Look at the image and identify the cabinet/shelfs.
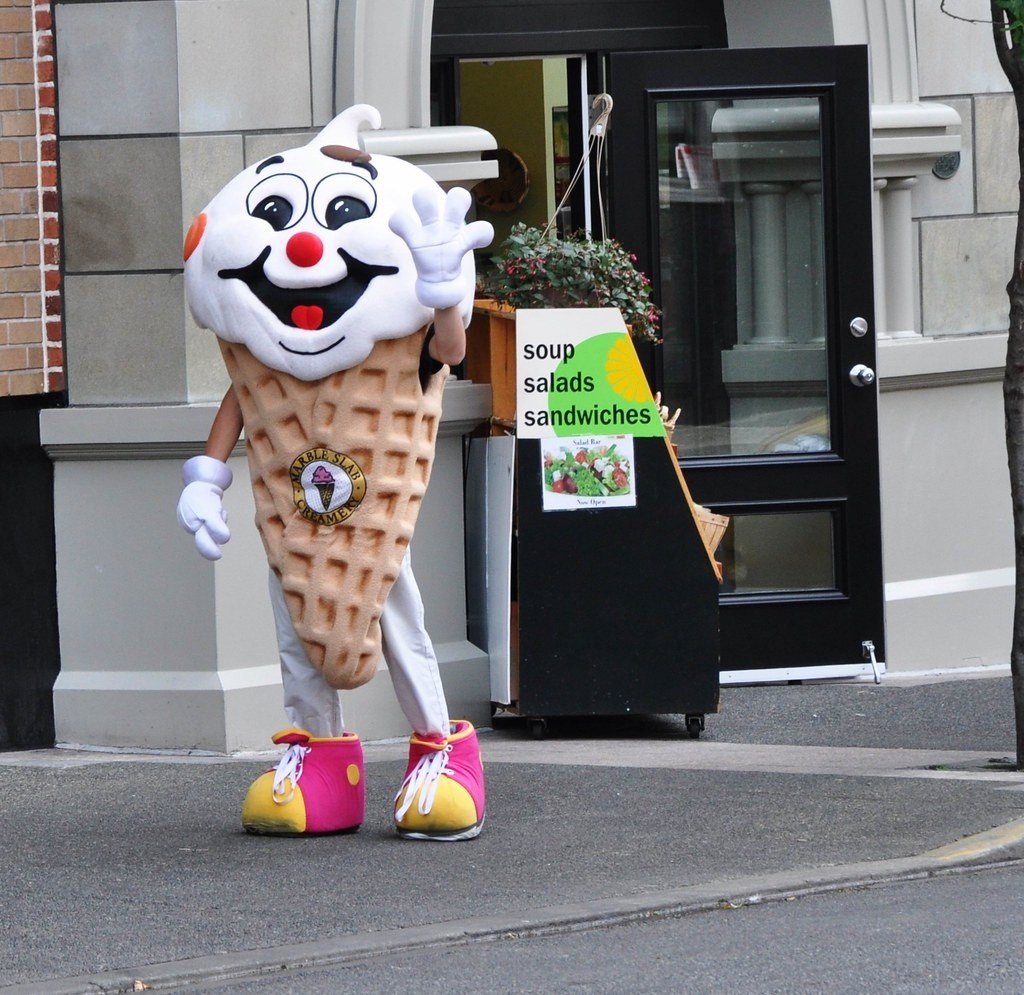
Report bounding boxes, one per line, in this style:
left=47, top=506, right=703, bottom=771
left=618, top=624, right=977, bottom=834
left=470, top=297, right=731, bottom=742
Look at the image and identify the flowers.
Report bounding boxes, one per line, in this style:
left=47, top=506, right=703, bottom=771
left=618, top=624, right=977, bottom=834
left=488, top=223, right=663, bottom=344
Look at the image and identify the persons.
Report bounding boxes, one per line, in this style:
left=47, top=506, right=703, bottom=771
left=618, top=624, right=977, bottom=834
left=174, top=100, right=497, bottom=845
